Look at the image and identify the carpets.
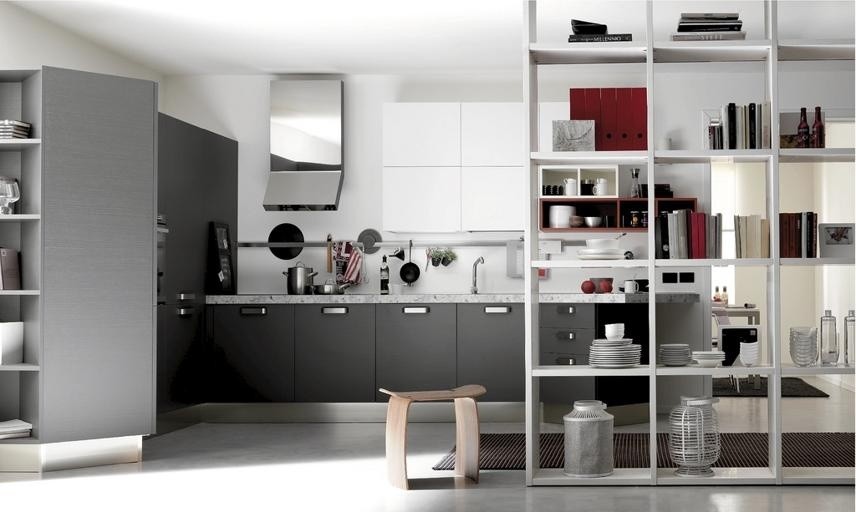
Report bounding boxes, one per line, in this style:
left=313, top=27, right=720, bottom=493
left=713, top=377, right=830, bottom=398
left=431, top=433, right=854, bottom=471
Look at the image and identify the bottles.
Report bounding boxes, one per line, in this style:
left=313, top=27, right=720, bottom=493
left=630, top=211, right=639, bottom=226
left=844, top=310, right=855, bottom=367
left=798, top=107, right=809, bottom=149
left=722, top=287, right=728, bottom=307
left=812, top=107, right=825, bottom=149
left=629, top=168, right=641, bottom=199
left=661, top=211, right=669, bottom=225
left=820, top=310, right=838, bottom=367
left=714, top=286, right=722, bottom=302
left=641, top=211, right=648, bottom=228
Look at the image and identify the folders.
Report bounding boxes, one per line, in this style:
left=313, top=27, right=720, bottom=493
left=632, top=88, right=647, bottom=150
left=616, top=88, right=632, bottom=150
left=570, top=89, right=585, bottom=120
left=585, top=89, right=601, bottom=150
left=601, top=88, right=616, bottom=150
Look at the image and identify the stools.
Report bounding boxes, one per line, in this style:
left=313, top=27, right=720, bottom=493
left=378, top=384, right=487, bottom=490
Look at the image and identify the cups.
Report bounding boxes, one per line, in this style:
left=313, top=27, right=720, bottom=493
left=565, top=179, right=577, bottom=196
left=593, top=184, right=609, bottom=196
left=624, top=281, right=639, bottom=294
left=596, top=178, right=607, bottom=185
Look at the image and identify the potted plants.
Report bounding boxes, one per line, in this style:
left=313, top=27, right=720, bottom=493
left=441, top=249, right=457, bottom=267
left=431, top=250, right=445, bottom=266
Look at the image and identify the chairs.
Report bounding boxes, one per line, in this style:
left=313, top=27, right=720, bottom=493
left=711, top=307, right=741, bottom=393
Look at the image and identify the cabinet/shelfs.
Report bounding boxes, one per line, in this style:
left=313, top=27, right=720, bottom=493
left=538, top=303, right=595, bottom=403
left=539, top=198, right=697, bottom=232
left=525, top=1, right=855, bottom=486
left=375, top=304, right=523, bottom=403
left=539, top=165, right=618, bottom=198
left=213, top=304, right=375, bottom=403
left=0, top=66, right=156, bottom=473
left=156, top=111, right=237, bottom=412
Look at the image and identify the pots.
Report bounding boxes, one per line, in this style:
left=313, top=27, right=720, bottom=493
left=311, top=282, right=350, bottom=296
left=282, top=261, right=317, bottom=294
left=400, top=241, right=421, bottom=285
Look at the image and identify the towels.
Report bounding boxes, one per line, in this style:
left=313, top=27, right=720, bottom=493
left=333, top=241, right=364, bottom=286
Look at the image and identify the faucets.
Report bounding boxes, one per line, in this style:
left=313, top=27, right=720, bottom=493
left=471, top=256, right=485, bottom=294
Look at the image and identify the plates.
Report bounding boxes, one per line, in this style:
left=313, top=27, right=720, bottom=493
left=579, top=254, right=625, bottom=260
left=692, top=351, right=726, bottom=367
left=588, top=339, right=641, bottom=368
left=659, top=343, right=691, bottom=366
left=577, top=248, right=625, bottom=255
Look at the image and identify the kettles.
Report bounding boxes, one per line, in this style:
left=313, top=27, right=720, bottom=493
left=1, top=176, right=22, bottom=215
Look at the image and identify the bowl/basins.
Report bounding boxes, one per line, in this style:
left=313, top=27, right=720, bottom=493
left=584, top=216, right=603, bottom=227
left=586, top=239, right=621, bottom=248
left=569, top=216, right=583, bottom=226
left=603, top=324, right=624, bottom=339
left=790, top=326, right=818, bottom=367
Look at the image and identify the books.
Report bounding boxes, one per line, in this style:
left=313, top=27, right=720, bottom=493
left=672, top=12, right=746, bottom=41
left=0, top=119, right=31, bottom=139
left=778, top=212, right=818, bottom=259
left=654, top=208, right=771, bottom=260
left=707, top=102, right=771, bottom=150
left=0, top=419, right=32, bottom=440
left=569, top=34, right=633, bottom=42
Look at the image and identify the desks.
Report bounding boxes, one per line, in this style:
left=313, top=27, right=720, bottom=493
left=714, top=308, right=760, bottom=325
